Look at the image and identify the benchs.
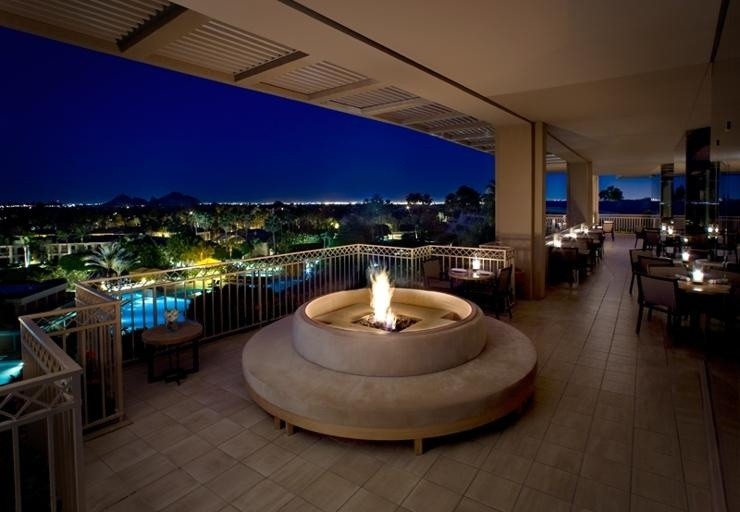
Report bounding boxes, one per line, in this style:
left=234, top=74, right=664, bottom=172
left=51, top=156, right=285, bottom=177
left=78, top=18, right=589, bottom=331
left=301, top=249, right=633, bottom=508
left=242, top=311, right=538, bottom=456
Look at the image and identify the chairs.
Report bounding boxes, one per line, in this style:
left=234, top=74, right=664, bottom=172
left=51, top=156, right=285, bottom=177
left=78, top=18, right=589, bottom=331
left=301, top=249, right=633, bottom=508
left=546, top=220, right=739, bottom=347
left=420, top=255, right=514, bottom=320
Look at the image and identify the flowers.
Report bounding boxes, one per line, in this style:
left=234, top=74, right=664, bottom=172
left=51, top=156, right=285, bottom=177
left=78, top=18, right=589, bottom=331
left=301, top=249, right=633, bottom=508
left=164, top=310, right=179, bottom=322
